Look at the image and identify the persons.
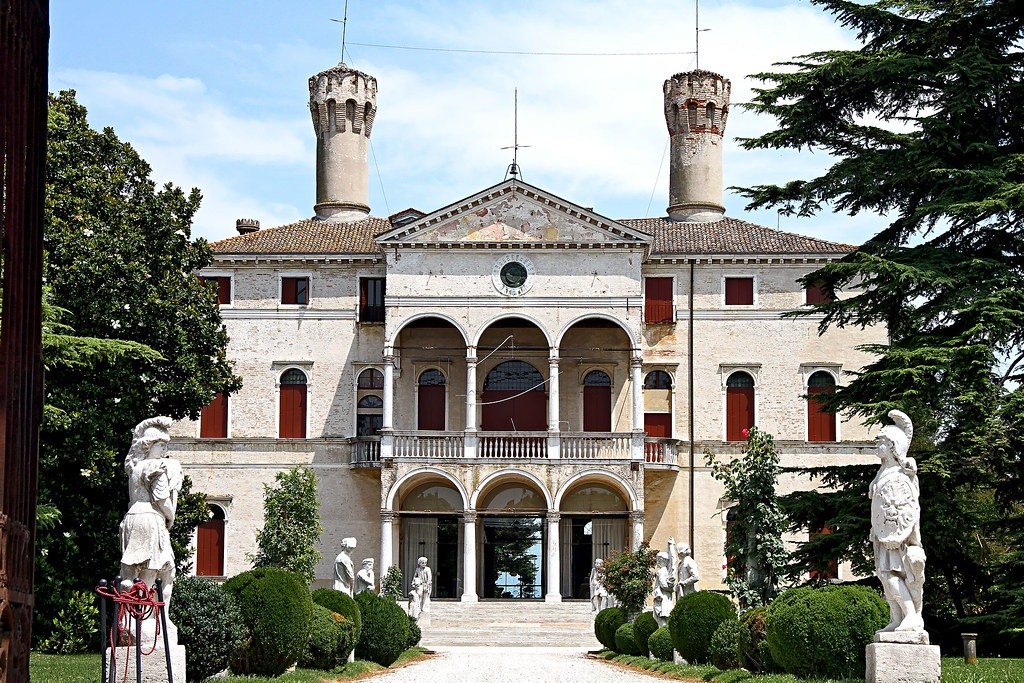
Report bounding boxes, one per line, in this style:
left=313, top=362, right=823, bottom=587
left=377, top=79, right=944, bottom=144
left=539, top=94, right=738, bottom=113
left=589, top=559, right=607, bottom=614
left=119, top=417, right=184, bottom=630
left=869, top=426, right=922, bottom=630
left=332, top=538, right=357, bottom=662
left=412, top=557, right=432, bottom=611
left=356, top=558, right=376, bottom=594
left=676, top=543, right=699, bottom=600
left=653, top=551, right=672, bottom=627
